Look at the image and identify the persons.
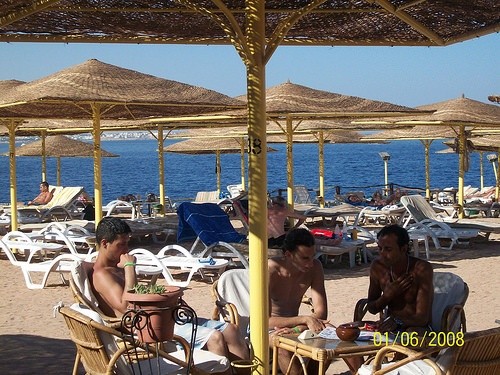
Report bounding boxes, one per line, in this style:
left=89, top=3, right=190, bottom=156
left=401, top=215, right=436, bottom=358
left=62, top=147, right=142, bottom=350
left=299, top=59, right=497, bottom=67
left=268, top=207, right=306, bottom=248
left=367, top=223, right=433, bottom=335
left=24, top=182, right=53, bottom=205
left=88, top=216, right=251, bottom=375
left=345, top=194, right=389, bottom=211
left=267, top=228, right=330, bottom=375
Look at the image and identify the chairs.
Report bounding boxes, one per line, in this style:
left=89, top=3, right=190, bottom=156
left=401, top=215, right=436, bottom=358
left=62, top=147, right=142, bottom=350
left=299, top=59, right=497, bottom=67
left=211, top=269, right=313, bottom=375
left=354, top=271, right=469, bottom=337
left=0, top=183, right=500, bottom=290
left=357, top=325, right=500, bottom=375
left=52, top=260, right=232, bottom=375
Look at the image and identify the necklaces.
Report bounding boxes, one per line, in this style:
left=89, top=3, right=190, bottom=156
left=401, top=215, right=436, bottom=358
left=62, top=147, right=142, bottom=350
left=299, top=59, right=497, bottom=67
left=390, top=253, right=409, bottom=281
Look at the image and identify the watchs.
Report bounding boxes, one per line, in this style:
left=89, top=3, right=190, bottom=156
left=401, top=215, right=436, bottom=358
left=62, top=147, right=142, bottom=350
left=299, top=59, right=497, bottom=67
left=394, top=316, right=405, bottom=331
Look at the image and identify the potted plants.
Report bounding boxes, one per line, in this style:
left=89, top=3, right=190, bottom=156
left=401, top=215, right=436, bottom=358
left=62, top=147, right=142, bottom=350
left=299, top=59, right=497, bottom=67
left=124, top=283, right=184, bottom=342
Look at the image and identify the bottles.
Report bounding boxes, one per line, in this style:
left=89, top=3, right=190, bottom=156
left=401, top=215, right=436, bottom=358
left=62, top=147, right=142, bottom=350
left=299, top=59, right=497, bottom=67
left=335, top=224, right=340, bottom=240
left=221, top=192, right=224, bottom=199
left=336, top=324, right=360, bottom=342
left=356, top=248, right=362, bottom=265
left=343, top=222, right=347, bottom=240
left=352, top=227, right=357, bottom=240
left=375, top=217, right=381, bottom=224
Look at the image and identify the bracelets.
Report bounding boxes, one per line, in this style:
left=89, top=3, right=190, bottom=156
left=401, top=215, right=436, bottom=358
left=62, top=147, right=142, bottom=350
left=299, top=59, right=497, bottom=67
left=294, top=326, right=300, bottom=333
left=123, top=261, right=136, bottom=266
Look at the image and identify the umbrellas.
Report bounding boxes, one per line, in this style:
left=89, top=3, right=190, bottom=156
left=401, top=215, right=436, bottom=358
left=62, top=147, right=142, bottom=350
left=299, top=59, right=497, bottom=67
left=0, top=0, right=500, bottom=375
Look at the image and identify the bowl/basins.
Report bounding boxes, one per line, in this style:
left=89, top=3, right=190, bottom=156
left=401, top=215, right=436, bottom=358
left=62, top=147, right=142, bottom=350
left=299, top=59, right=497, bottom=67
left=380, top=221, right=385, bottom=224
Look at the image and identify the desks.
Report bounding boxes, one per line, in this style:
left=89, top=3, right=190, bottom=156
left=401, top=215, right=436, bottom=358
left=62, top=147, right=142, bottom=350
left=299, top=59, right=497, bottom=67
left=273, top=330, right=430, bottom=375
left=131, top=200, right=160, bottom=220
